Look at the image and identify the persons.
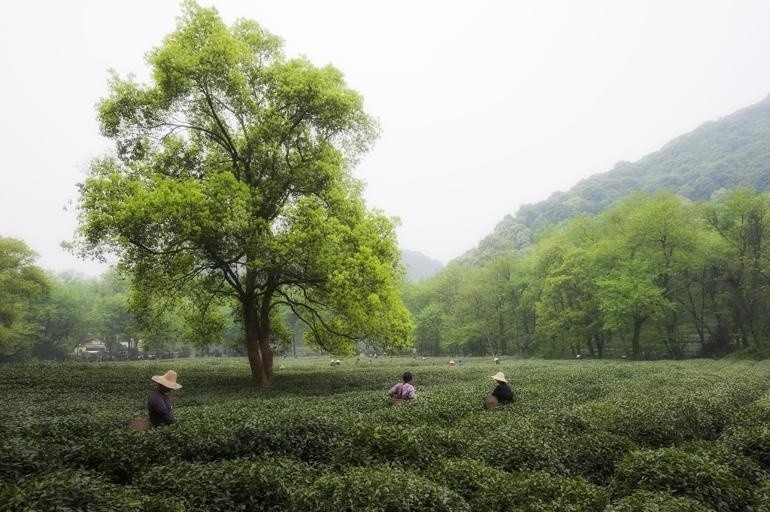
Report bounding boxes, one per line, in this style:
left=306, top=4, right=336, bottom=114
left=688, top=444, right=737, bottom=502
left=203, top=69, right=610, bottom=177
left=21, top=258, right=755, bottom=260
left=388, top=371, right=418, bottom=400
left=323, top=348, right=585, bottom=366
left=490, top=372, right=514, bottom=404
left=147, top=370, right=181, bottom=428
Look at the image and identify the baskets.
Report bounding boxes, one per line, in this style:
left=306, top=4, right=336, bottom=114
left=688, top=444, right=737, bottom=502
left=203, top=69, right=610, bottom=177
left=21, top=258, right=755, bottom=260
left=485, top=397, right=499, bottom=409
left=387, top=398, right=406, bottom=407
left=129, top=419, right=152, bottom=432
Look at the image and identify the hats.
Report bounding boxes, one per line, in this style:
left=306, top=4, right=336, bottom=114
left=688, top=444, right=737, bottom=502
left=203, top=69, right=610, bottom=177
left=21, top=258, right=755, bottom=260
left=491, top=372, right=507, bottom=382
left=151, top=370, right=182, bottom=390
